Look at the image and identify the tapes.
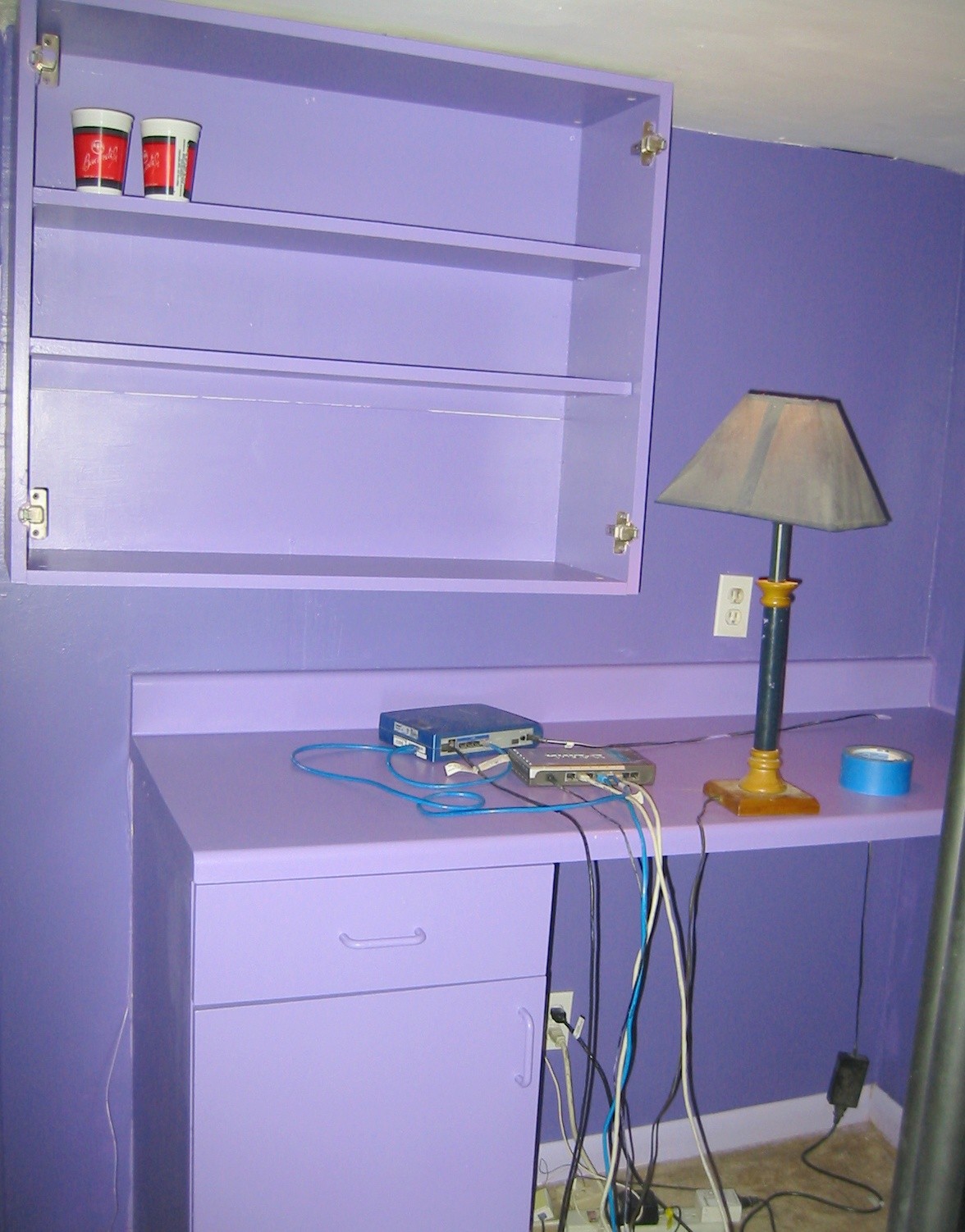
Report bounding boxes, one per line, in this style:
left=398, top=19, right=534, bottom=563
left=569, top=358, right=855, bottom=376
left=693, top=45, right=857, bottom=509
left=838, top=743, right=915, bottom=798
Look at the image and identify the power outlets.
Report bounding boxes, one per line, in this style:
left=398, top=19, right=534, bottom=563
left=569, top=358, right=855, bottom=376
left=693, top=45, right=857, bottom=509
left=713, top=573, right=753, bottom=638
left=546, top=991, right=573, bottom=1050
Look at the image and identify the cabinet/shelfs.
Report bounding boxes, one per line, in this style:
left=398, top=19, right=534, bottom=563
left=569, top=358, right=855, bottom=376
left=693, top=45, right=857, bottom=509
left=0, top=0, right=674, bottom=594
left=193, top=864, right=555, bottom=1231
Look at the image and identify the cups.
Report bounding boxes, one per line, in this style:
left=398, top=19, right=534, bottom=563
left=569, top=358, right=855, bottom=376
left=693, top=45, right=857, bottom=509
left=141, top=117, right=203, bottom=201
left=71, top=107, right=134, bottom=196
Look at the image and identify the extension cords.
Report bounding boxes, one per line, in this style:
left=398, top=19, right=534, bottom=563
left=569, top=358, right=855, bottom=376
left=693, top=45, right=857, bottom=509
left=563, top=1207, right=727, bottom=1232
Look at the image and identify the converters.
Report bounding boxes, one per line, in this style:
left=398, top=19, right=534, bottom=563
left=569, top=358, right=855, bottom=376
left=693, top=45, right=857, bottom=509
left=827, top=1052, right=869, bottom=1106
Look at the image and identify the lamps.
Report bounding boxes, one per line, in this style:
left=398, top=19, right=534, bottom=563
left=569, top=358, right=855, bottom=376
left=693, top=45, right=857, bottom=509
left=654, top=392, right=891, bottom=819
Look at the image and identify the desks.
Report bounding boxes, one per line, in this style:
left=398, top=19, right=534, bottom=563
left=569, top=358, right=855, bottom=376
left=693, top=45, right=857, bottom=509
left=132, top=708, right=965, bottom=1232
